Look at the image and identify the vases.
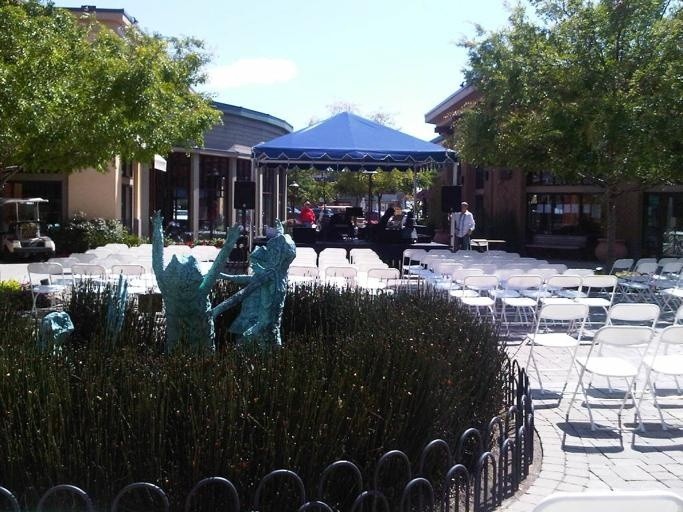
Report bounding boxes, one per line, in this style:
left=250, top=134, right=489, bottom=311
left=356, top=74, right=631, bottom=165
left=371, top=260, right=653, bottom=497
left=592, top=236, right=628, bottom=263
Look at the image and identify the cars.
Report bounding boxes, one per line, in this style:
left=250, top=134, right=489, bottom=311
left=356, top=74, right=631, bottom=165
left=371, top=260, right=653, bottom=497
left=545, top=223, right=607, bottom=238
left=172, top=210, right=188, bottom=221
left=235, top=205, right=422, bottom=227
left=661, top=230, right=682, bottom=257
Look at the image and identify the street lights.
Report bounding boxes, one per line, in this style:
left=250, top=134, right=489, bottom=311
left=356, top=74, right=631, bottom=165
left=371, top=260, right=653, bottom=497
left=287, top=180, right=300, bottom=218
left=361, top=170, right=378, bottom=213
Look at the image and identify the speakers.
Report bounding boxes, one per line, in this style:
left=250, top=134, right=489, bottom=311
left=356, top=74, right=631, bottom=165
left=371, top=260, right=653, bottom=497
left=441, top=185, right=462, bottom=212
left=234, top=182, right=255, bottom=209
left=292, top=228, right=316, bottom=244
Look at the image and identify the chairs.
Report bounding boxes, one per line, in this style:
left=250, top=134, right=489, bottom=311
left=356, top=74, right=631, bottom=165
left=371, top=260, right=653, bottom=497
left=287, top=247, right=399, bottom=298
left=400, top=247, right=546, bottom=336
left=24, top=241, right=221, bottom=300
left=525, top=258, right=683, bottom=432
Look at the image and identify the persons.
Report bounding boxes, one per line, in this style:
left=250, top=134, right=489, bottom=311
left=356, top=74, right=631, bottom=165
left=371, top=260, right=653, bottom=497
left=448, top=201, right=475, bottom=251
left=299, top=200, right=315, bottom=228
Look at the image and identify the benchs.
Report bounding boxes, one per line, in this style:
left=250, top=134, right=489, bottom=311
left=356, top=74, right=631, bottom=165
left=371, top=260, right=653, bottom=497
left=524, top=233, right=587, bottom=259
